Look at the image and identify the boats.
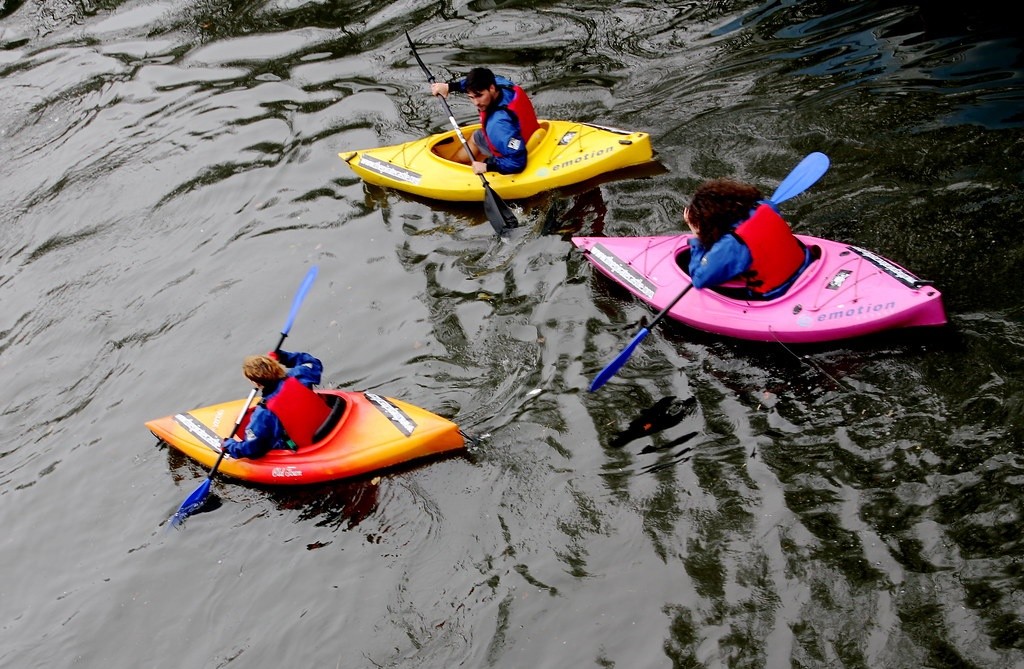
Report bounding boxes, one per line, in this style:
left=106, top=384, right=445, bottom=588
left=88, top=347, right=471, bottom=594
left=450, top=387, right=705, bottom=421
left=144, top=390, right=463, bottom=485
left=574, top=236, right=947, bottom=342
left=337, top=119, right=659, bottom=201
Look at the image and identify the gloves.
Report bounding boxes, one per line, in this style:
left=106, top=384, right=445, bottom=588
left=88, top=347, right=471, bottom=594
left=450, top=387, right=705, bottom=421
left=222, top=438, right=228, bottom=451
left=269, top=352, right=279, bottom=361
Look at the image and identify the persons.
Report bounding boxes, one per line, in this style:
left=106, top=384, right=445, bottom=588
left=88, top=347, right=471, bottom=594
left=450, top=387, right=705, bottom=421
left=219, top=350, right=332, bottom=460
left=432, top=68, right=540, bottom=176
left=683, top=180, right=810, bottom=301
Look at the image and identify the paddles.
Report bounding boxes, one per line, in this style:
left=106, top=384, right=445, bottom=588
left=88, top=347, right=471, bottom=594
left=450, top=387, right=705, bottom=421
left=165, top=265, right=318, bottom=534
left=587, top=150, right=831, bottom=392
left=403, top=27, right=520, bottom=234
